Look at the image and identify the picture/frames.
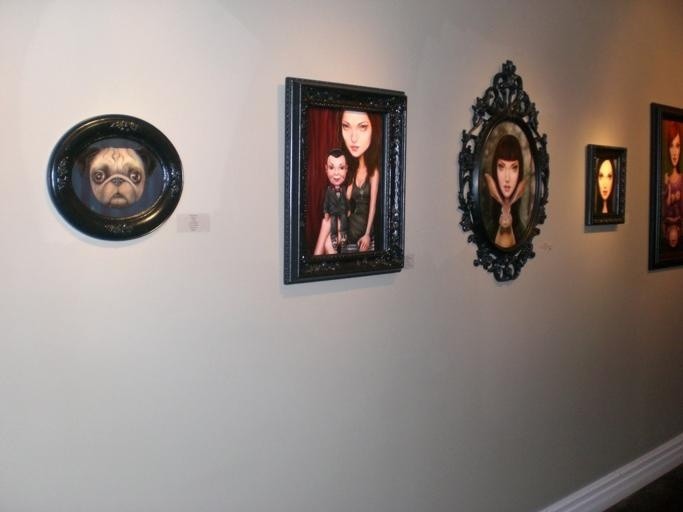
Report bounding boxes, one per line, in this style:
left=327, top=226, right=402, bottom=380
left=648, top=102, right=682, bottom=272
left=283, top=76, right=404, bottom=286
left=47, top=113, right=183, bottom=242
left=457, top=60, right=550, bottom=282
left=584, top=144, right=627, bottom=225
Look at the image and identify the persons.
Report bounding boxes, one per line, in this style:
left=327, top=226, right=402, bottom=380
left=663, top=123, right=683, bottom=248
left=322, top=147, right=352, bottom=248
left=596, top=159, right=616, bottom=213
left=314, top=110, right=379, bottom=255
left=485, top=134, right=530, bottom=249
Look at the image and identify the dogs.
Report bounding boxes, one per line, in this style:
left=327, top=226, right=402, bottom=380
left=75, top=143, right=158, bottom=209
